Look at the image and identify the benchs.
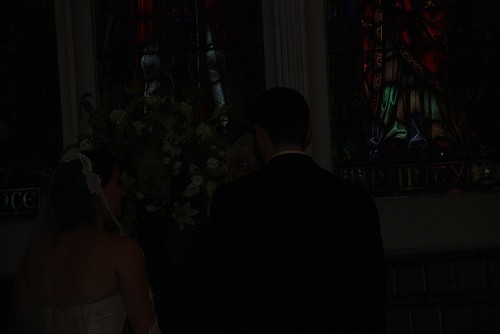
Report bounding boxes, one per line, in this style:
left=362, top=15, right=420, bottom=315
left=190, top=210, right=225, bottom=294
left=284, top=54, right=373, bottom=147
left=382, top=241, right=499, bottom=334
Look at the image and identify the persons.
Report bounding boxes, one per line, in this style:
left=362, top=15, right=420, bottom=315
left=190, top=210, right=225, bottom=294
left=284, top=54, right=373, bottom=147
left=199, top=86, right=387, bottom=334
left=13, top=149, right=161, bottom=334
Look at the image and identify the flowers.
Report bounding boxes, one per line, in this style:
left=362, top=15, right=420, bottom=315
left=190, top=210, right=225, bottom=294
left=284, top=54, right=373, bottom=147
left=66, top=89, right=241, bottom=236
